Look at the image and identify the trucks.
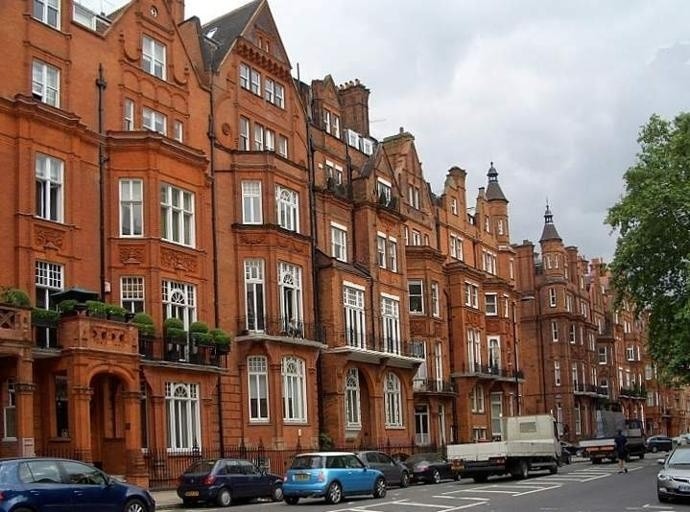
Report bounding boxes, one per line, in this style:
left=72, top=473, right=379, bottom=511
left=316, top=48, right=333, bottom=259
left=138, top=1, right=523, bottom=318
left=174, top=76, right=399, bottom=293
left=579, top=419, right=646, bottom=463
left=445, top=413, right=569, bottom=481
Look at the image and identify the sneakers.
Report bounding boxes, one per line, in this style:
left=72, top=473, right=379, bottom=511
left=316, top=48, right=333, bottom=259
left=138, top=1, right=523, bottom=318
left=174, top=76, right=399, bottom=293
left=618, top=467, right=627, bottom=473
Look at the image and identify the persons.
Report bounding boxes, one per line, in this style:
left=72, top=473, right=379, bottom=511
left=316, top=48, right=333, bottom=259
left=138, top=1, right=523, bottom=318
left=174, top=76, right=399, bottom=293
left=614, top=428, right=630, bottom=474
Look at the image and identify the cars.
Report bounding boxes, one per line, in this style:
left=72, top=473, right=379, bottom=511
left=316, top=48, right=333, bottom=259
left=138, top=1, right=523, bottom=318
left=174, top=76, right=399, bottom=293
left=656, top=441, right=690, bottom=504
left=1, top=456, right=158, bottom=512
left=280, top=451, right=461, bottom=503
left=644, top=433, right=690, bottom=453
left=178, top=457, right=285, bottom=507
left=559, top=447, right=572, bottom=466
